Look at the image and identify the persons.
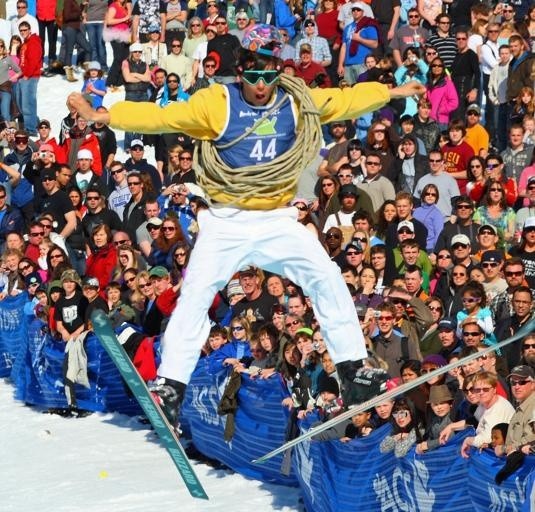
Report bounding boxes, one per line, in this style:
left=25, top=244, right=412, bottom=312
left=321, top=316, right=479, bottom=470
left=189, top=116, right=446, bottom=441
left=293, top=151, right=535, bottom=457
left=0, top=246, right=182, bottom=418
left=0, top=169, right=191, bottom=285
left=155, top=266, right=305, bottom=469
left=276, top=1, right=534, bottom=198
left=66, top=23, right=427, bottom=406
left=104, top=1, right=295, bottom=238
left=1, top=1, right=117, bottom=218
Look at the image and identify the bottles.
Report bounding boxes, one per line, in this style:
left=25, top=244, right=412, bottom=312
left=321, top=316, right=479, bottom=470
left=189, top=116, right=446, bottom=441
left=241, top=23, right=286, bottom=58
left=426, top=385, right=454, bottom=404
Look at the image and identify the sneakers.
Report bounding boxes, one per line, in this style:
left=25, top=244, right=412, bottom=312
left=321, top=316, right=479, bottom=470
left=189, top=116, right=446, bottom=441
left=146, top=375, right=187, bottom=438
left=336, top=359, right=390, bottom=407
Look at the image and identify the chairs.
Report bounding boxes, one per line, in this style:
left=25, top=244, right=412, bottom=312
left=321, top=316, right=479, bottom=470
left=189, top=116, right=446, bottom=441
left=146, top=375, right=187, bottom=438
left=336, top=359, right=390, bottom=407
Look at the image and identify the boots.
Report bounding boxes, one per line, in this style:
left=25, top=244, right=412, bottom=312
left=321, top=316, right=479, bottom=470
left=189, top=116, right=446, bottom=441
left=62, top=66, right=77, bottom=82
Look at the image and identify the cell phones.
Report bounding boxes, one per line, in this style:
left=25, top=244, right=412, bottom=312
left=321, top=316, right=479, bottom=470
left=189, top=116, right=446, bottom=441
left=373, top=310, right=381, bottom=317
left=312, top=342, right=320, bottom=351
left=173, top=184, right=185, bottom=194
left=38, top=152, right=47, bottom=159
left=409, top=56, right=418, bottom=62
left=502, top=3, right=509, bottom=9
left=7, top=129, right=15, bottom=133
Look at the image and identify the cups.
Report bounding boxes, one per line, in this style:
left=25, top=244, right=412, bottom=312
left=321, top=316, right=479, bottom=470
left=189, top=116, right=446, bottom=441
left=238, top=69, right=278, bottom=85
left=392, top=410, right=410, bottom=419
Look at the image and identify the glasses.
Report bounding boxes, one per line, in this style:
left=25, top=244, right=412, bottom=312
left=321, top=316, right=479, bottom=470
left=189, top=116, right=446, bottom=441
left=1, top=6, right=245, bottom=330
left=392, top=410, right=410, bottom=419
left=284, top=8, right=535, bottom=395
left=238, top=69, right=278, bottom=85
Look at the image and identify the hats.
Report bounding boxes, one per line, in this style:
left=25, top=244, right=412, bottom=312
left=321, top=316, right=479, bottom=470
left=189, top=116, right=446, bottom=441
left=241, top=23, right=286, bottom=58
left=331, top=121, right=345, bottom=126
left=345, top=242, right=362, bottom=250
left=146, top=217, right=163, bottom=227
left=129, top=139, right=144, bottom=147
left=506, top=365, right=533, bottom=381
left=82, top=276, right=99, bottom=287
left=481, top=250, right=501, bottom=262
left=300, top=43, right=311, bottom=52
left=426, top=385, right=454, bottom=404
left=148, top=266, right=167, bottom=278
left=86, top=61, right=101, bottom=70
left=523, top=217, right=533, bottom=228
left=397, top=221, right=414, bottom=232
left=379, top=109, right=393, bottom=122
left=450, top=234, right=470, bottom=248
left=339, top=184, right=359, bottom=198
left=466, top=104, right=480, bottom=114
left=478, top=224, right=497, bottom=236
left=77, top=149, right=93, bottom=160
left=37, top=119, right=49, bottom=128
left=436, top=317, right=456, bottom=329
left=39, top=144, right=53, bottom=152
